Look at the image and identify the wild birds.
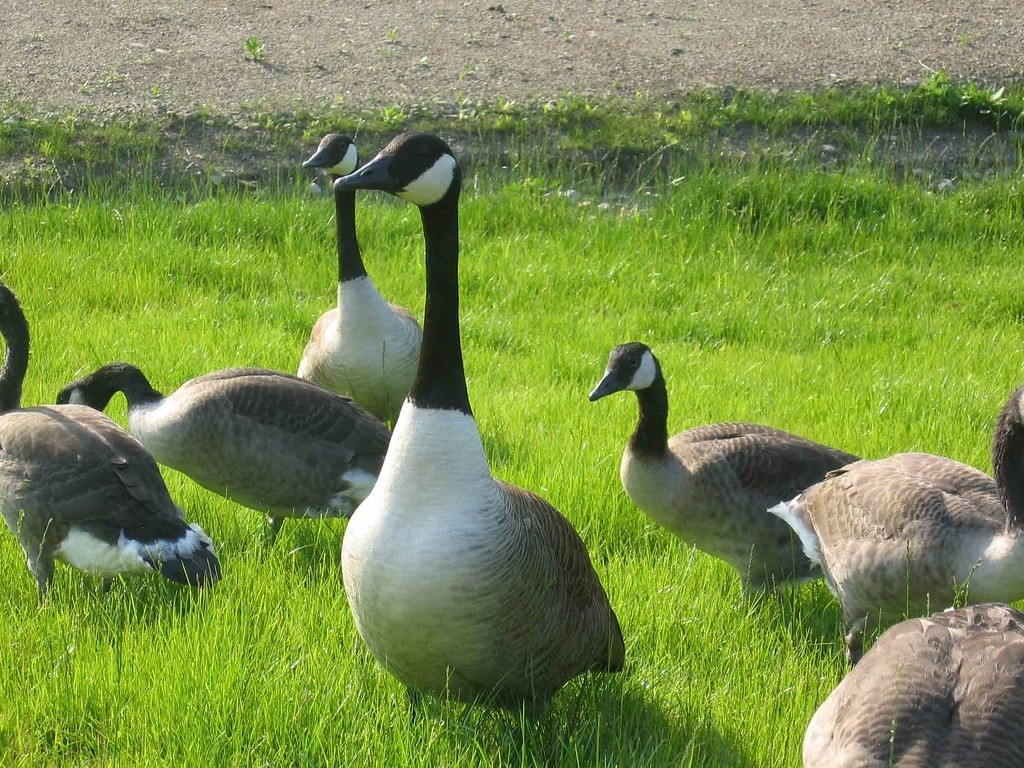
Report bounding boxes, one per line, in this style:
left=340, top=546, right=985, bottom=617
left=589, top=342, right=863, bottom=606
left=54, top=360, right=392, bottom=554
left=803, top=603, right=1024, bottom=768
left=331, top=131, right=626, bottom=708
left=763, top=385, right=1024, bottom=670
left=299, top=132, right=423, bottom=429
left=1, top=281, right=224, bottom=596
